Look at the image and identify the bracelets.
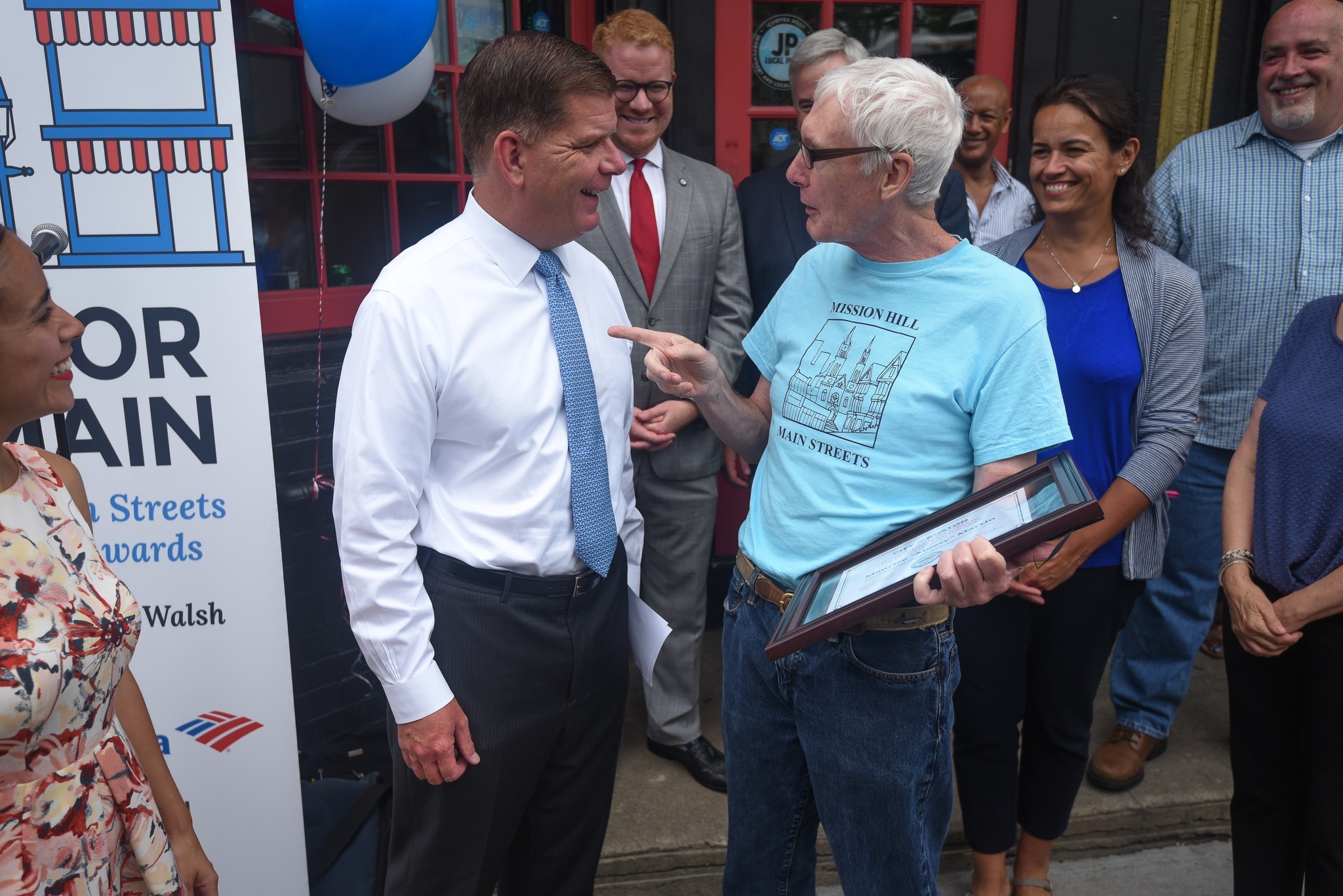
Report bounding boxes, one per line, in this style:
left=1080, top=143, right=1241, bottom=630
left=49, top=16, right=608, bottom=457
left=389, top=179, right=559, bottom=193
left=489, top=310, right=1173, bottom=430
left=1218, top=557, right=1255, bottom=580
left=1218, top=548, right=1255, bottom=569
left=1219, top=561, right=1254, bottom=587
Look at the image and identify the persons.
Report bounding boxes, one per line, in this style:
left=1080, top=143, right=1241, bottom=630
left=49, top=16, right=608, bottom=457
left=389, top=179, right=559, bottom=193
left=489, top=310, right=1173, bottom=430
left=952, top=74, right=1204, bottom=896
left=726, top=27, right=973, bottom=487
left=1086, top=0, right=1343, bottom=790
left=950, top=74, right=1037, bottom=247
left=1, top=225, right=218, bottom=895
left=329, top=33, right=645, bottom=896
left=611, top=57, right=1075, bottom=895
left=1218, top=293, right=1343, bottom=895
left=573, top=9, right=755, bottom=794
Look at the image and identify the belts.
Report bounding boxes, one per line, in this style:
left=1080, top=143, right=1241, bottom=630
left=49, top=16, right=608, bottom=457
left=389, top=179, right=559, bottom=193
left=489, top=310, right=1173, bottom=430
left=416, top=549, right=615, bottom=601
left=732, top=550, right=949, bottom=630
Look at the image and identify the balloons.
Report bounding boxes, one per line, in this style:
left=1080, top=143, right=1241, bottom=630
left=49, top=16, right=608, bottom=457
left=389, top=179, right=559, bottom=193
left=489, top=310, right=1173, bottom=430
left=293, top=0, right=439, bottom=87
left=302, top=37, right=436, bottom=124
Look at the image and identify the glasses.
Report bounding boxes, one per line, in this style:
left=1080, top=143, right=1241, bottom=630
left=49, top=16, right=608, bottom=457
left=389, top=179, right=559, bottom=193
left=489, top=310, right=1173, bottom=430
left=615, top=79, right=673, bottom=104
left=796, top=139, right=880, bottom=170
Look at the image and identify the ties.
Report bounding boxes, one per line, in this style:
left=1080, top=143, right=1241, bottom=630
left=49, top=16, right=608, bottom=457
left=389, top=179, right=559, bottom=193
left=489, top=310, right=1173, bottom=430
left=629, top=158, right=661, bottom=302
left=534, top=251, right=618, bottom=580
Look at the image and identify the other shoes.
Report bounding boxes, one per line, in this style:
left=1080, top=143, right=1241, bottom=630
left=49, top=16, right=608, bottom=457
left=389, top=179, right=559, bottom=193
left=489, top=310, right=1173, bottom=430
left=1201, top=634, right=1225, bottom=660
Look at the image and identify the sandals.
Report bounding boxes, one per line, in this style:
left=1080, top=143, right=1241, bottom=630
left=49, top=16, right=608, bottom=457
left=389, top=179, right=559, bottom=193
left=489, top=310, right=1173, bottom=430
left=1012, top=877, right=1053, bottom=896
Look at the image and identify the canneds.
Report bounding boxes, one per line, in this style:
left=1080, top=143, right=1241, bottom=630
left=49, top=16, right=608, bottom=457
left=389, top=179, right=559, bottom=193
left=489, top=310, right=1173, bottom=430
left=330, top=265, right=354, bottom=286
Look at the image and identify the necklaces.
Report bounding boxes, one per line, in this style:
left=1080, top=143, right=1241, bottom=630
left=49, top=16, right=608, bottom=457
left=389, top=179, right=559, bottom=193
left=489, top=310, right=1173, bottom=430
left=1041, top=233, right=1114, bottom=294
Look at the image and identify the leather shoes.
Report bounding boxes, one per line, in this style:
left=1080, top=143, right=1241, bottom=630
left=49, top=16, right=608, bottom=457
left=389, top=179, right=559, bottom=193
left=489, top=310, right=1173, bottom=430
left=648, top=736, right=727, bottom=793
left=1087, top=725, right=1167, bottom=791
